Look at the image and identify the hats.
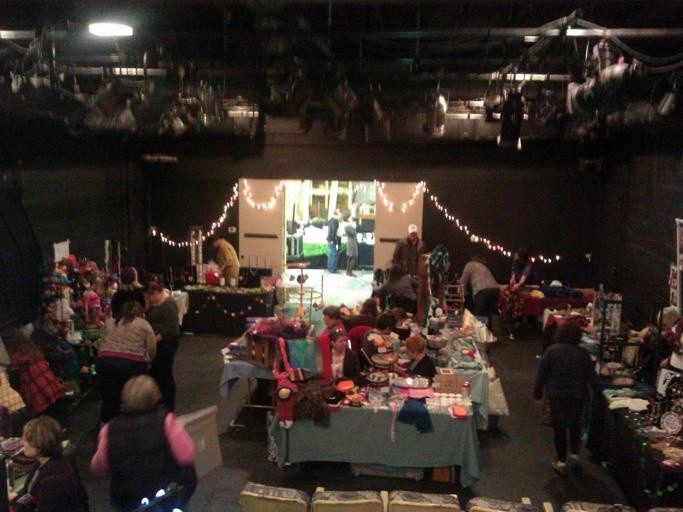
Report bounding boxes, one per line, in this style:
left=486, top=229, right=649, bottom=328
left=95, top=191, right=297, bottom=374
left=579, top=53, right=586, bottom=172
left=408, top=223, right=418, bottom=233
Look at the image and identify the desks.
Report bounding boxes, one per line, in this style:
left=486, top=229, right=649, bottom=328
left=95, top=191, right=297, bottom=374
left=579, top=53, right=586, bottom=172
left=1, top=236, right=683, bottom=511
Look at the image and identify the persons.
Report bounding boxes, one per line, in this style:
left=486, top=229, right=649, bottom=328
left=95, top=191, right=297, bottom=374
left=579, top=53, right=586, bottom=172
left=286, top=210, right=359, bottom=277
left=533, top=321, right=603, bottom=476
left=206, top=235, right=240, bottom=286
left=253, top=225, right=501, bottom=390
left=1, top=268, right=196, bottom=511
left=633, top=326, right=673, bottom=388
left=510, top=247, right=536, bottom=291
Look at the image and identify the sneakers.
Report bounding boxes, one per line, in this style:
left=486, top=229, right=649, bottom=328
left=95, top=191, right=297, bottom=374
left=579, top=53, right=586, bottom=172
left=552, top=460, right=567, bottom=476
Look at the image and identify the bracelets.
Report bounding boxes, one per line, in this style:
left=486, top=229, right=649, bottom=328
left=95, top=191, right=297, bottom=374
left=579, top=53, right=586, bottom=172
left=518, top=282, right=523, bottom=288
